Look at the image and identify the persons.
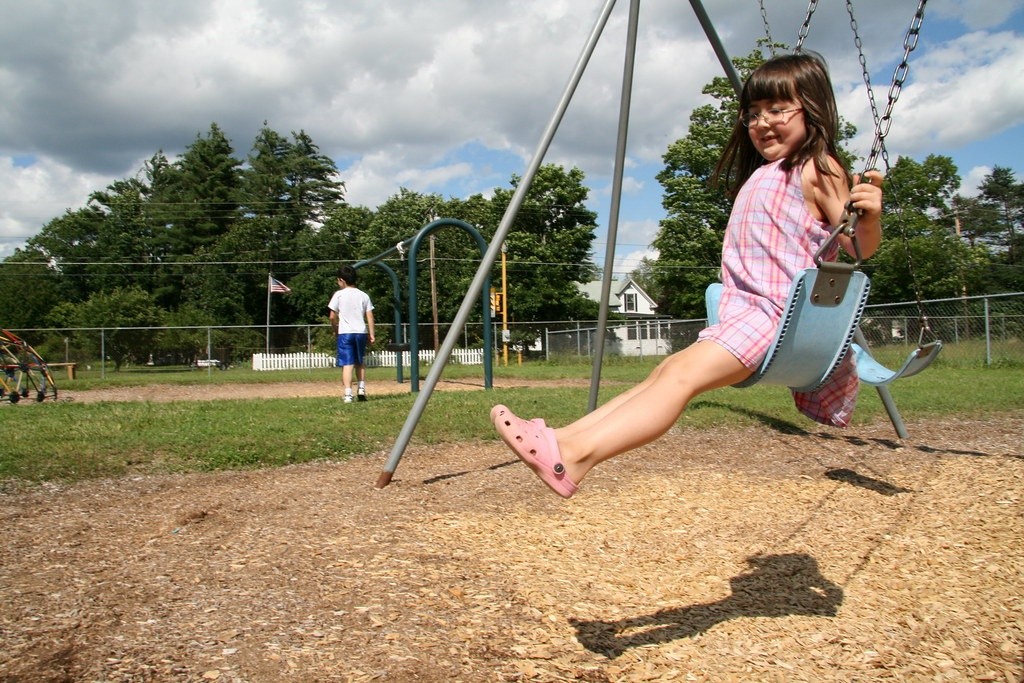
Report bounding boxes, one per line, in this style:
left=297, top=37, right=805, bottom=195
left=328, top=266, right=375, bottom=403
left=491, top=55, right=883, bottom=498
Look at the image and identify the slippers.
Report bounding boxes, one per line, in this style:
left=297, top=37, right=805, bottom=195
left=491, top=404, right=578, bottom=499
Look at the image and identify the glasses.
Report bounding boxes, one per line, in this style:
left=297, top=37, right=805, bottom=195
left=738, top=108, right=803, bottom=128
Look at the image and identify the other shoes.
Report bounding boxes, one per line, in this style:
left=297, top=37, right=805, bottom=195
left=357, top=387, right=369, bottom=400
left=344, top=395, right=355, bottom=404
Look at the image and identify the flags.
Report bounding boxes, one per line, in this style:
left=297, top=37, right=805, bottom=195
left=270, top=278, right=290, bottom=292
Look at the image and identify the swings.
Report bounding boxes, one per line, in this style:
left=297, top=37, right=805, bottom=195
left=702, top=0, right=932, bottom=395
left=384, top=241, right=423, bottom=352
left=756, top=0, right=947, bottom=388
